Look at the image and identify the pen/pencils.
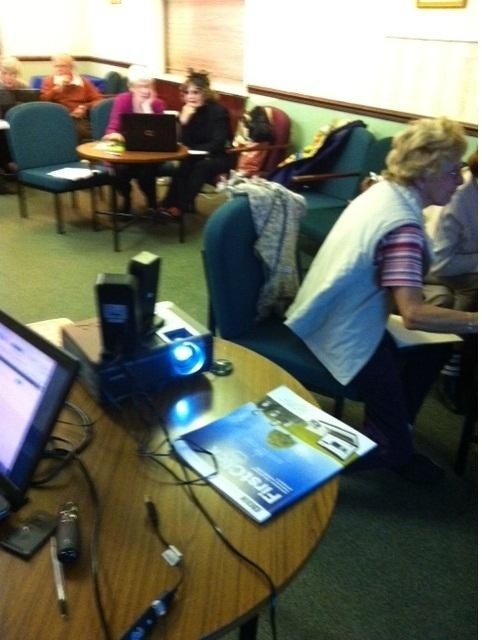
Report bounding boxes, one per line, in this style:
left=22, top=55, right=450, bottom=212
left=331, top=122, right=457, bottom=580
left=50, top=536, right=67, bottom=619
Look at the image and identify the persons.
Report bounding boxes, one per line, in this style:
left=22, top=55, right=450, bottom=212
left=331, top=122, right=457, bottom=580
left=422, top=150, right=478, bottom=417
left=38, top=52, right=104, bottom=139
left=253, top=109, right=477, bottom=514
left=155, top=72, right=236, bottom=225
left=0, top=55, right=30, bottom=89
left=103, top=64, right=164, bottom=213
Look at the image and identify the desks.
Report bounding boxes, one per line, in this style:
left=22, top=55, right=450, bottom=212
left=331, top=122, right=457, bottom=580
left=75, top=139, right=189, bottom=252
left=2, top=317, right=345, bottom=638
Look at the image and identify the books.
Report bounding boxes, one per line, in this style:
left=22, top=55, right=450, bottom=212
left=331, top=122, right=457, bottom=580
left=166, top=382, right=379, bottom=525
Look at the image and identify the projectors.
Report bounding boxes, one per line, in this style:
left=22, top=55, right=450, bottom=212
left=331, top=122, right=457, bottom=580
left=61, top=299, right=216, bottom=408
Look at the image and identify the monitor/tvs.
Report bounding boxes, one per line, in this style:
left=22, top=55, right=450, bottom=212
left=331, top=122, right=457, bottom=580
left=0, top=306, right=84, bottom=510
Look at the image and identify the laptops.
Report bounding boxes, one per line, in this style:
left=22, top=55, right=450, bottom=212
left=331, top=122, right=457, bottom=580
left=117, top=112, right=182, bottom=154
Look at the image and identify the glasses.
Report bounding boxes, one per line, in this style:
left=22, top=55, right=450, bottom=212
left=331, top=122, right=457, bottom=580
left=442, top=161, right=468, bottom=180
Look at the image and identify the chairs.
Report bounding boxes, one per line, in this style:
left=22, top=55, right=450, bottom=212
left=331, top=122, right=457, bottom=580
left=291, top=125, right=395, bottom=286
left=224, top=105, right=289, bottom=183
left=4, top=100, right=109, bottom=234
left=199, top=192, right=361, bottom=420
left=89, top=98, right=176, bottom=200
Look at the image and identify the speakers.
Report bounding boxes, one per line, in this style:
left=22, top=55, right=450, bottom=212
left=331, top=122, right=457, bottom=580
left=95, top=271, right=145, bottom=355
left=129, top=251, right=165, bottom=335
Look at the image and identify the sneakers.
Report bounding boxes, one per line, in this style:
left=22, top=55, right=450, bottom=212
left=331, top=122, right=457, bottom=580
left=147, top=206, right=181, bottom=219
